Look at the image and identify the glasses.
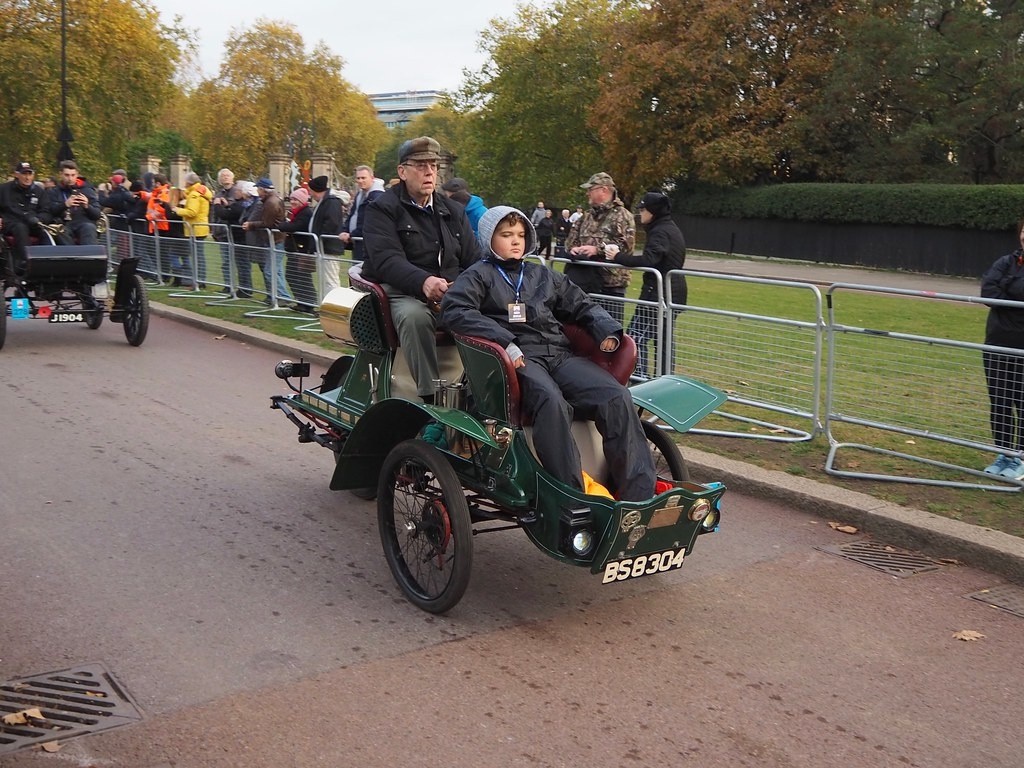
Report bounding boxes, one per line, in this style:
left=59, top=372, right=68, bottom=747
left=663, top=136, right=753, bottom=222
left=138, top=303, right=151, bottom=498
left=403, top=162, right=441, bottom=171
left=589, top=186, right=602, bottom=191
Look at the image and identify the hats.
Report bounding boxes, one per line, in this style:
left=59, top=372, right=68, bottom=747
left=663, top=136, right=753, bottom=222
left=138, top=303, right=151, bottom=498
left=252, top=179, right=274, bottom=189
left=14, top=163, right=33, bottom=173
left=398, top=136, right=442, bottom=164
left=579, top=173, right=615, bottom=189
left=112, top=169, right=127, bottom=176
left=290, top=188, right=310, bottom=205
left=184, top=173, right=201, bottom=184
left=635, top=194, right=671, bottom=217
left=441, top=178, right=468, bottom=193
left=309, top=176, right=328, bottom=191
left=130, top=180, right=145, bottom=192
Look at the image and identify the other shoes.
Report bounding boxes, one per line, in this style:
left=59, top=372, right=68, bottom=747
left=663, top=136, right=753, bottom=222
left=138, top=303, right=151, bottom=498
left=220, top=287, right=230, bottom=293
left=172, top=277, right=182, bottom=286
left=235, top=289, right=253, bottom=297
left=294, top=303, right=315, bottom=313
left=199, top=283, right=206, bottom=289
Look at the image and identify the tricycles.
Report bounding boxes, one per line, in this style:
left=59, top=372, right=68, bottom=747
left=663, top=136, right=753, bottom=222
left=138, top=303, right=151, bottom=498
left=270, top=259, right=729, bottom=616
left=0, top=213, right=151, bottom=352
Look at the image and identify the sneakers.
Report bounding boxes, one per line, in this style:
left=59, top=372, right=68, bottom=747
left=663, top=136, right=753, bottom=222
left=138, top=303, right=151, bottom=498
left=984, top=453, right=1013, bottom=475
left=1000, top=459, right=1024, bottom=480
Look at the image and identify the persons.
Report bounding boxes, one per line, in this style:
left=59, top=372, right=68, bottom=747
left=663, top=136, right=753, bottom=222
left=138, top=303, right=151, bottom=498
left=603, top=192, right=686, bottom=382
left=564, top=172, right=636, bottom=325
left=530, top=202, right=583, bottom=262
left=441, top=205, right=658, bottom=507
left=280, top=166, right=490, bottom=316
left=362, top=137, right=497, bottom=437
left=981, top=219, right=1024, bottom=481
left=98, top=168, right=291, bottom=305
left=0, top=159, right=101, bottom=273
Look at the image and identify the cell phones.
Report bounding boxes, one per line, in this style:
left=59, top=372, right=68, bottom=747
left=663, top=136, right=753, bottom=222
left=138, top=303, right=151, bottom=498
left=153, top=199, right=160, bottom=203
left=72, top=189, right=80, bottom=195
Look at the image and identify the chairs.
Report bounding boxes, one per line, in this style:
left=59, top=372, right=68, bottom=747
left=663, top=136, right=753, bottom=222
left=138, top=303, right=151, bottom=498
left=450, top=323, right=637, bottom=430
left=349, top=262, right=458, bottom=351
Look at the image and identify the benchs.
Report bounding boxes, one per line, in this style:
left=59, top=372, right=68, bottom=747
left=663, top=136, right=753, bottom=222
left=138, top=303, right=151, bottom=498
left=0, top=210, right=108, bottom=247
